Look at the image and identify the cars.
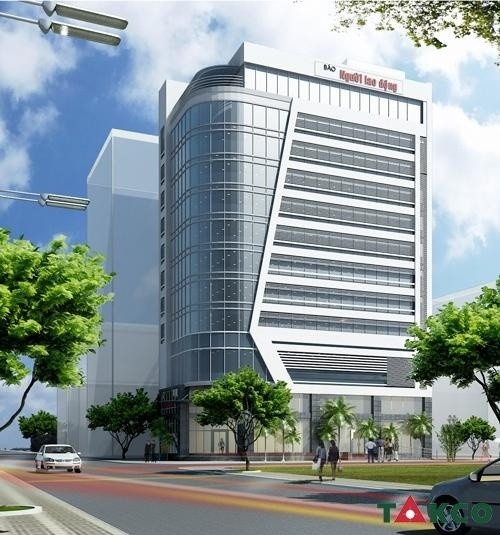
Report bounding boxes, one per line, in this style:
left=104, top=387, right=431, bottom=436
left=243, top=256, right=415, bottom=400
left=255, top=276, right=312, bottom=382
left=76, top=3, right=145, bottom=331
left=34, top=444, right=82, bottom=474
left=426, top=457, right=499, bottom=534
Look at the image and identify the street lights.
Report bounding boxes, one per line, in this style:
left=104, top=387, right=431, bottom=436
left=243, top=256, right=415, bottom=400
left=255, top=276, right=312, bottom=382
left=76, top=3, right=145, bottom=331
left=0, top=1, right=128, bottom=46
left=1, top=190, right=92, bottom=214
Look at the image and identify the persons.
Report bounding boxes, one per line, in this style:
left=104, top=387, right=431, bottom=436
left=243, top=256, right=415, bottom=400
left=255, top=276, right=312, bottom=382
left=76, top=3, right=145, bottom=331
left=316, top=440, right=327, bottom=480
left=365, top=437, right=399, bottom=463
left=327, top=440, right=341, bottom=480
left=144, top=440, right=151, bottom=461
left=151, top=440, right=157, bottom=462
